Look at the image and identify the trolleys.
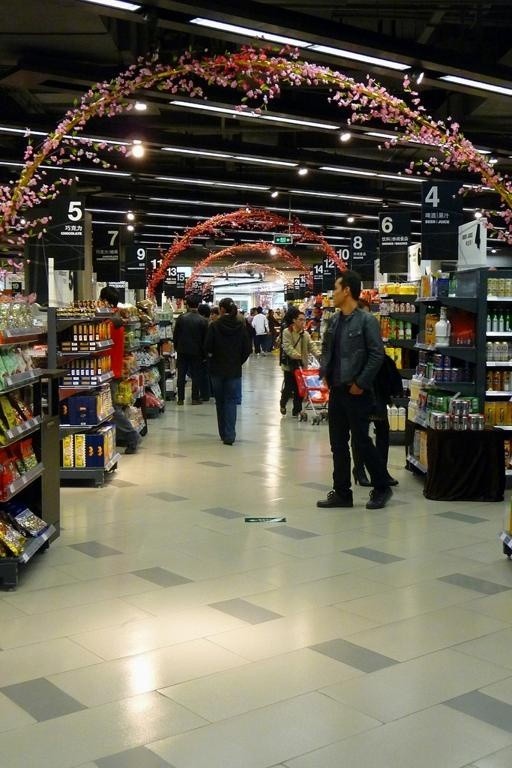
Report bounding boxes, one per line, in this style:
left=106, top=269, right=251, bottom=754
left=296, top=353, right=330, bottom=426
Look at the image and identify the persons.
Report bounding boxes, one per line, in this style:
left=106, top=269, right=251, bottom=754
left=316, top=269, right=395, bottom=510
left=280, top=312, right=322, bottom=416
left=350, top=297, right=400, bottom=488
left=171, top=292, right=282, bottom=405
left=204, top=297, right=254, bottom=446
left=98, top=285, right=144, bottom=454
left=281, top=306, right=303, bottom=398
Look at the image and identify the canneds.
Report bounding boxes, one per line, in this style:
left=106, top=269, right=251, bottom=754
left=493, top=341, right=501, bottom=361
left=429, top=398, right=485, bottom=430
left=486, top=342, right=493, bottom=361
left=502, top=370, right=510, bottom=391
left=394, top=302, right=415, bottom=313
left=416, top=350, right=458, bottom=382
left=487, top=279, right=512, bottom=297
left=508, top=342, right=512, bottom=361
left=427, top=391, right=480, bottom=425
left=501, top=342, right=508, bottom=361
left=486, top=371, right=493, bottom=390
left=493, top=370, right=502, bottom=390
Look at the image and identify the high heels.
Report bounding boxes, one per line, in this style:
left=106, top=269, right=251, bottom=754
left=386, top=470, right=398, bottom=486
left=353, top=467, right=370, bottom=486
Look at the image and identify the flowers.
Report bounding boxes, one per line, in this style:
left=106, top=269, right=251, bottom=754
left=0, top=43, right=512, bottom=285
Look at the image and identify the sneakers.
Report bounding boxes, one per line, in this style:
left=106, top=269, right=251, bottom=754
left=192, top=400, right=202, bottom=404
left=125, top=436, right=142, bottom=454
left=177, top=400, right=184, bottom=405
left=366, top=486, right=393, bottom=509
left=317, top=490, right=353, bottom=507
left=281, top=407, right=286, bottom=414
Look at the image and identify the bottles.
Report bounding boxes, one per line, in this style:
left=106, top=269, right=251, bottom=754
left=424, top=305, right=511, bottom=346
